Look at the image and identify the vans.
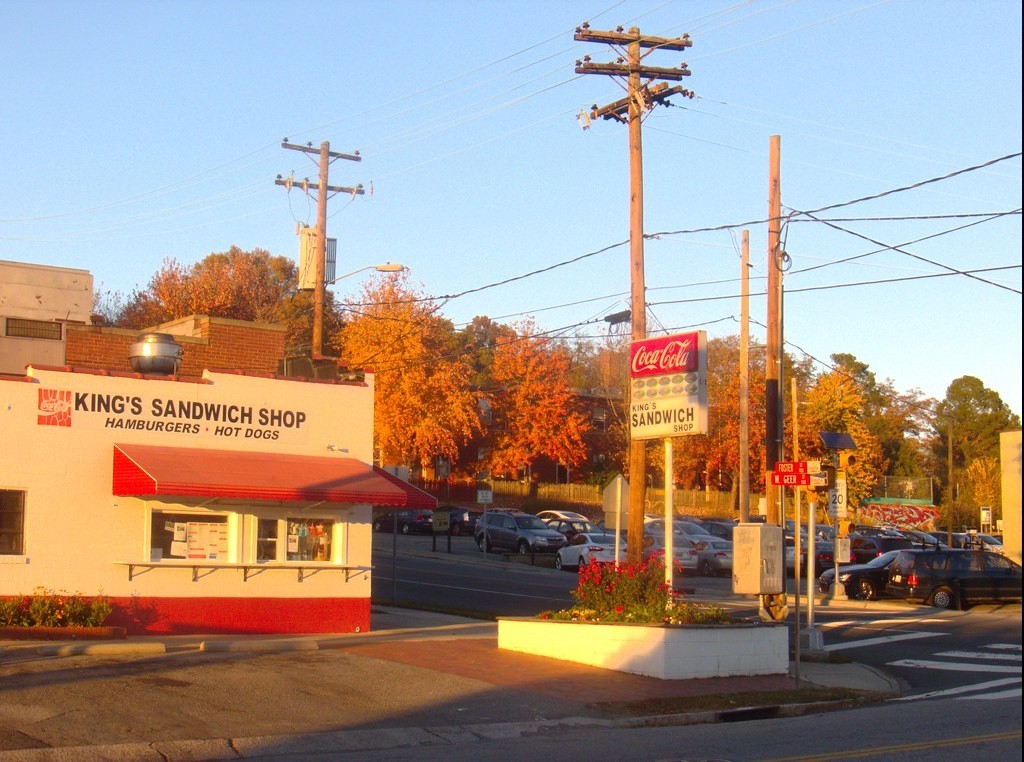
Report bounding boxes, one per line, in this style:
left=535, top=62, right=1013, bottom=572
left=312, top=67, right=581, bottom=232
left=488, top=507, right=525, bottom=516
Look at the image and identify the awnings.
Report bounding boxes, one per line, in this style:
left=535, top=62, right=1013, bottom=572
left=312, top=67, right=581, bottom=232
left=113, top=443, right=408, bottom=507
left=372, top=465, right=439, bottom=511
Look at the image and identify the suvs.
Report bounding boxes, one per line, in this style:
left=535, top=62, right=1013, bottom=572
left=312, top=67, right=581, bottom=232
left=473, top=511, right=568, bottom=553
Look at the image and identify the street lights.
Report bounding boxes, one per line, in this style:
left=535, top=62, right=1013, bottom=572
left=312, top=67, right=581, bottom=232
left=310, top=264, right=407, bottom=363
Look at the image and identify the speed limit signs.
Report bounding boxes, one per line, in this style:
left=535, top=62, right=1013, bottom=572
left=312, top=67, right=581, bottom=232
left=828, top=479, right=848, bottom=516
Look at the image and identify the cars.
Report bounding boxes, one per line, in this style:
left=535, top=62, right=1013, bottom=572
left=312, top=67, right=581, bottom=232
left=554, top=531, right=628, bottom=575
left=884, top=548, right=1023, bottom=608
left=598, top=511, right=1004, bottom=575
left=534, top=510, right=591, bottom=531
left=818, top=548, right=930, bottom=601
left=428, top=506, right=486, bottom=536
left=372, top=506, right=441, bottom=534
left=543, top=517, right=605, bottom=542
